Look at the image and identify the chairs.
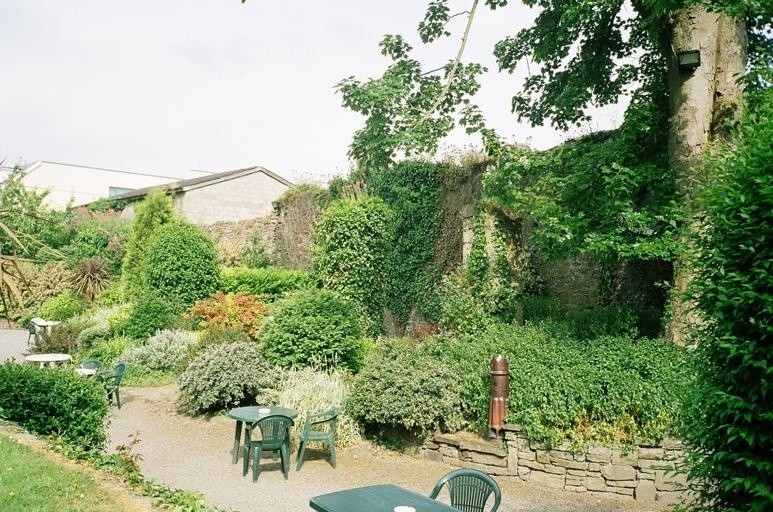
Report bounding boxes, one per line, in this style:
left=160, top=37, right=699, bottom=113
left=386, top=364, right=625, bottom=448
left=79, top=359, right=125, bottom=410
left=429, top=466, right=502, bottom=512
left=243, top=406, right=339, bottom=482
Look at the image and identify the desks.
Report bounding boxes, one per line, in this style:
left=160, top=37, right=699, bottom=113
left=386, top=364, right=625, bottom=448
left=228, top=405, right=298, bottom=465
left=308, top=482, right=464, bottom=512
left=23, top=353, right=72, bottom=372
